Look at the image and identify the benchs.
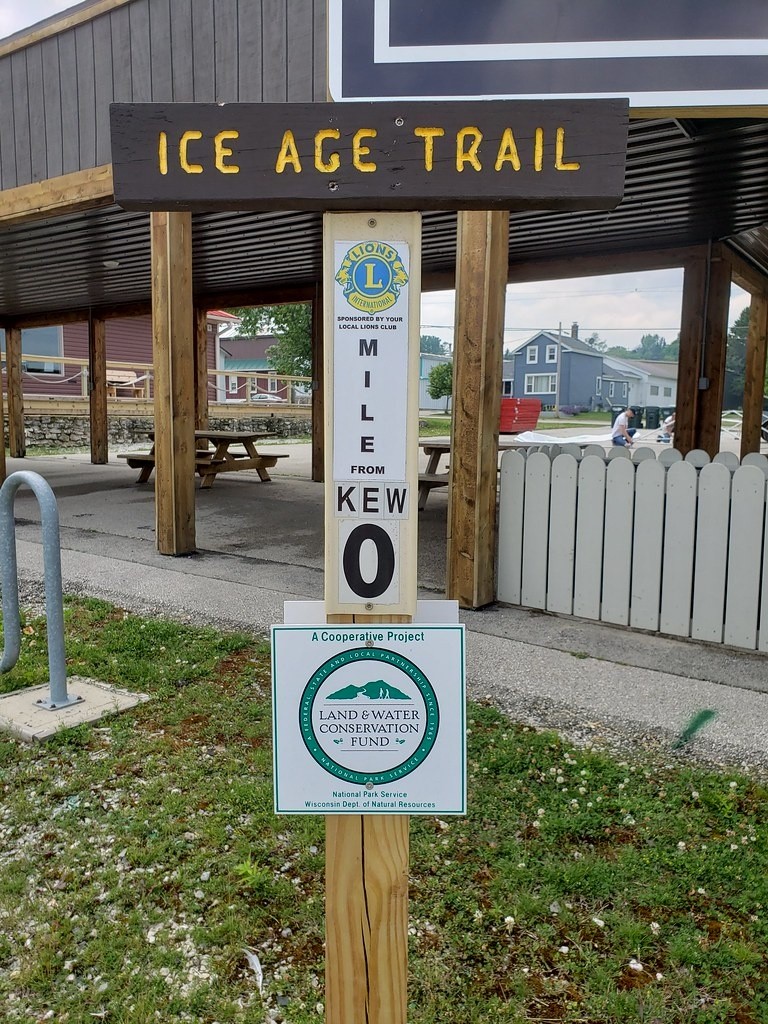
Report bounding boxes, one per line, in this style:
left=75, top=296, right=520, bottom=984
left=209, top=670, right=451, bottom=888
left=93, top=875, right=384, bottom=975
left=106, top=369, right=145, bottom=398
left=417, top=473, right=450, bottom=484
left=117, top=454, right=225, bottom=488
left=195, top=449, right=289, bottom=482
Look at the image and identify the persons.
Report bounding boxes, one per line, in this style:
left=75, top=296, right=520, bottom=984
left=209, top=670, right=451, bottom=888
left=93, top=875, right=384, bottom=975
left=611, top=408, right=637, bottom=450
left=656, top=412, right=676, bottom=443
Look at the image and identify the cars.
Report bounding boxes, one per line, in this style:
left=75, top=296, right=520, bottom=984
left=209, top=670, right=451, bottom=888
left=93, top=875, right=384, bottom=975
left=243, top=392, right=283, bottom=404
left=762, top=396, right=768, bottom=443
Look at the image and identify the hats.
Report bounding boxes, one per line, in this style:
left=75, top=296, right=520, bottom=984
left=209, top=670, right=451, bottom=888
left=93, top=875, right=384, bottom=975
left=630, top=406, right=638, bottom=415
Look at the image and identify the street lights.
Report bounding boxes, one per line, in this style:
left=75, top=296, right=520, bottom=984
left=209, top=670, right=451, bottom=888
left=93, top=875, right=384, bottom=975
left=442, top=342, right=452, bottom=362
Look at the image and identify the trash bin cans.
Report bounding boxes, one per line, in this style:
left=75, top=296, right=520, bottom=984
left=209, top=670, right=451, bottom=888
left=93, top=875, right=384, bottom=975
left=610, top=404, right=627, bottom=429
left=661, top=405, right=675, bottom=422
left=645, top=406, right=661, bottom=429
left=628, top=405, right=644, bottom=427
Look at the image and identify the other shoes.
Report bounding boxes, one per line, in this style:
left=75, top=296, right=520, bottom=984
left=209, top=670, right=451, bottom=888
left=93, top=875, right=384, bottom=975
left=657, top=435, right=662, bottom=442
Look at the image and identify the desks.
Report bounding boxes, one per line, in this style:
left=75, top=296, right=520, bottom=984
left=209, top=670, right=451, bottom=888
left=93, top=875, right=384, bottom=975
left=131, top=430, right=278, bottom=488
left=418, top=439, right=588, bottom=511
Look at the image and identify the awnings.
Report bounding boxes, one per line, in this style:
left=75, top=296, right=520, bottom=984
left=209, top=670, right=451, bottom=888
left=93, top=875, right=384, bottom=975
left=224, top=359, right=280, bottom=371
